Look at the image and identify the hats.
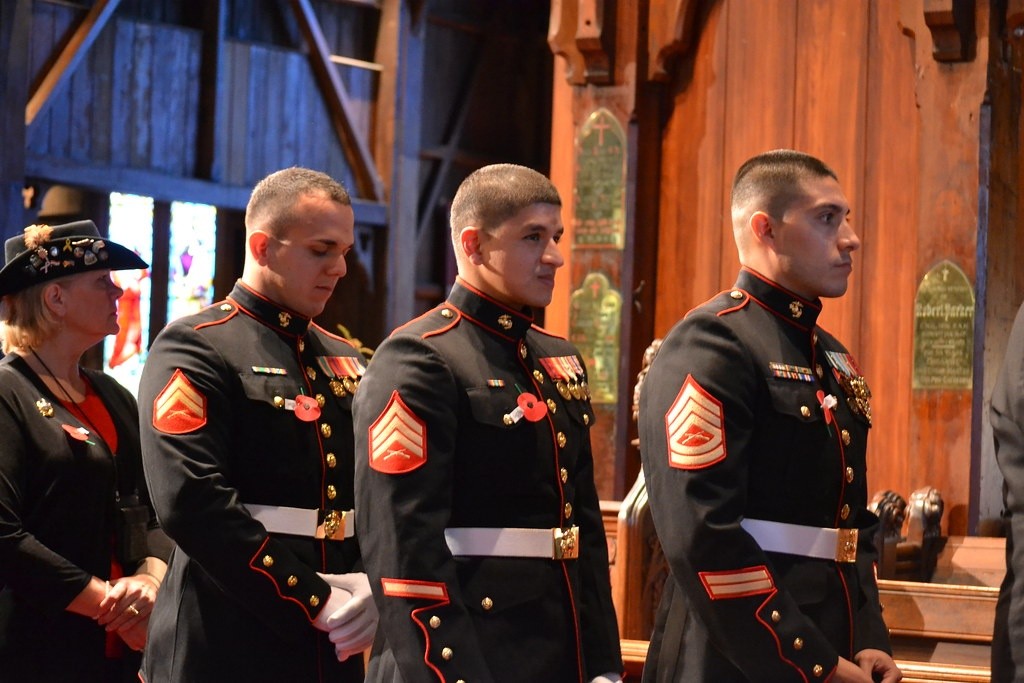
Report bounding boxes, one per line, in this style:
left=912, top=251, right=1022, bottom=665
left=0, top=219, right=149, bottom=298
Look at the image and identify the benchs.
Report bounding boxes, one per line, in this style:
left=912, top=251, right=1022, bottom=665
left=864, top=488, right=1007, bottom=682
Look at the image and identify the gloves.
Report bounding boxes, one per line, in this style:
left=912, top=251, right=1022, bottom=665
left=312, top=572, right=380, bottom=662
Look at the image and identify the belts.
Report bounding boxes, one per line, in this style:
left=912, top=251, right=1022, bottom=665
left=741, top=518, right=858, bottom=561
left=244, top=504, right=354, bottom=540
left=444, top=527, right=581, bottom=560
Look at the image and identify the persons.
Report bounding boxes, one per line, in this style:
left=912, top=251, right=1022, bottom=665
left=351, top=164, right=626, bottom=683
left=137, top=168, right=380, bottom=683
left=989, top=303, right=1024, bottom=683
left=1, top=221, right=174, bottom=683
left=638, top=150, right=904, bottom=682
